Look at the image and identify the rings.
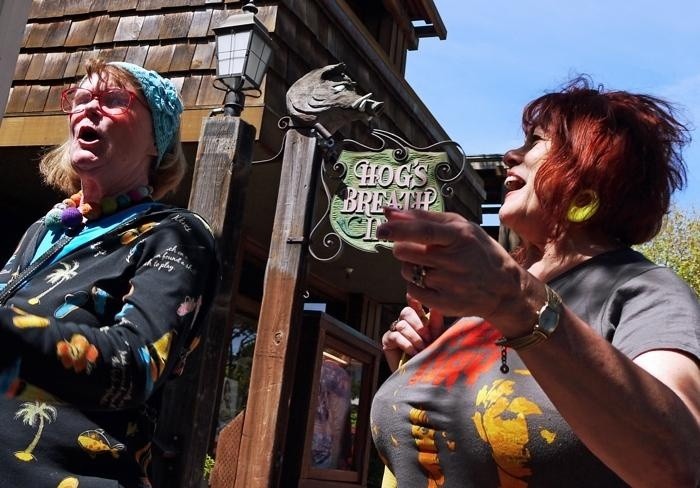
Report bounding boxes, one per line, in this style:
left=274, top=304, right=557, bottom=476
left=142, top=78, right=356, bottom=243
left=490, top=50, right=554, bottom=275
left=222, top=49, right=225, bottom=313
left=408, top=264, right=429, bottom=289
left=389, top=319, right=399, bottom=332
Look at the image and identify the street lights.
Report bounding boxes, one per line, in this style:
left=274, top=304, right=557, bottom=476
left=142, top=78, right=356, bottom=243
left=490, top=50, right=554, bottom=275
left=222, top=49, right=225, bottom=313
left=141, top=1, right=282, bottom=487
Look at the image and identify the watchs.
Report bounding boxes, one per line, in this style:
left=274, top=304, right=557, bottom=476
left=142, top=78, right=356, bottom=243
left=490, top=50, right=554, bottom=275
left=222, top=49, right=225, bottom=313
left=494, top=283, right=562, bottom=351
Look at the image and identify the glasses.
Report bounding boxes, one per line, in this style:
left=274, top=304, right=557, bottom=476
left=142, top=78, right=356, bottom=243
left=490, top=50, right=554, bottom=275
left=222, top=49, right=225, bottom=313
left=60, top=85, right=155, bottom=119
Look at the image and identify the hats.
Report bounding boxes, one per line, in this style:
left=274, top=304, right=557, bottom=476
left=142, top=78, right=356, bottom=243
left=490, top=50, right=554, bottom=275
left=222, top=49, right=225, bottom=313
left=103, top=61, right=184, bottom=170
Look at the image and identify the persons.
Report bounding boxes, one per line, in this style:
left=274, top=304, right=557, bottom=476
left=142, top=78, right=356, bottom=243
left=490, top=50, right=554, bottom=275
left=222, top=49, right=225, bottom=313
left=368, top=75, right=700, bottom=488
left=1, top=58, right=222, bottom=488
